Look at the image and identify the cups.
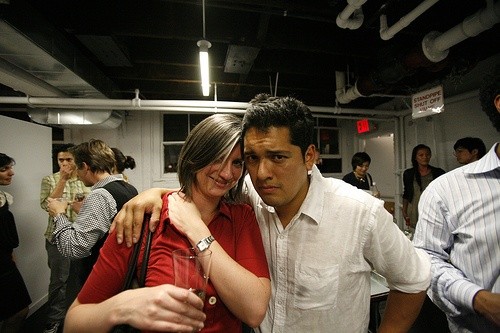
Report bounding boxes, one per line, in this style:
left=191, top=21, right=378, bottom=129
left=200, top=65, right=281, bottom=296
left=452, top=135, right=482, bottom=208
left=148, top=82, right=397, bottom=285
left=172, top=248, right=212, bottom=311
left=56, top=198, right=67, bottom=215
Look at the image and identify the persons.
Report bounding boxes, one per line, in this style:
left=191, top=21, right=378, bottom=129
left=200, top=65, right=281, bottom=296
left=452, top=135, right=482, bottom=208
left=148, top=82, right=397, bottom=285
left=402, top=144, right=446, bottom=234
left=454, top=137, right=487, bottom=164
left=109, top=93, right=432, bottom=333
left=45, top=138, right=139, bottom=314
left=40, top=143, right=91, bottom=333
left=342, top=152, right=380, bottom=198
left=0, top=153, right=32, bottom=333
left=64, top=114, right=271, bottom=333
left=111, top=148, right=136, bottom=182
left=412, top=67, right=500, bottom=333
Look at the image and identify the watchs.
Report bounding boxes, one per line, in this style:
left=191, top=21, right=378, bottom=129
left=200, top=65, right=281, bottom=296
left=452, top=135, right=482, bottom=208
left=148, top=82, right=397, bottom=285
left=193, top=235, right=216, bottom=255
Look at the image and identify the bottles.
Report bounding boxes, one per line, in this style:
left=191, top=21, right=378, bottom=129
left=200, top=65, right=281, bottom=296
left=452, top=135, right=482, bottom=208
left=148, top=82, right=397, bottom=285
left=371, top=182, right=377, bottom=197
left=404, top=217, right=413, bottom=241
left=75, top=180, right=85, bottom=201
